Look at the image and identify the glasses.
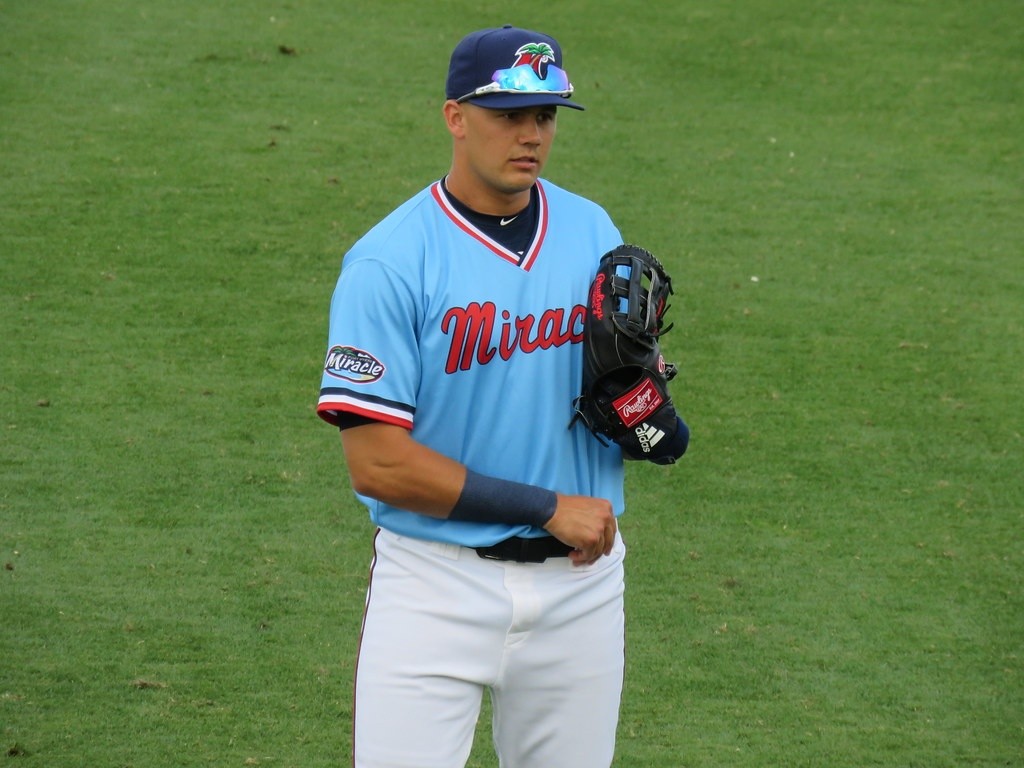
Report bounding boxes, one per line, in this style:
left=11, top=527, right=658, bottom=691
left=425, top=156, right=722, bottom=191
left=456, top=64, right=574, bottom=103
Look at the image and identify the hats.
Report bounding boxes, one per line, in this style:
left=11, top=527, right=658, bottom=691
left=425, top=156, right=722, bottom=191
left=446, top=25, right=586, bottom=111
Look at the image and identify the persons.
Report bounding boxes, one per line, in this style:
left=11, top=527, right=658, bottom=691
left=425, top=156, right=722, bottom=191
left=315, top=23, right=690, bottom=768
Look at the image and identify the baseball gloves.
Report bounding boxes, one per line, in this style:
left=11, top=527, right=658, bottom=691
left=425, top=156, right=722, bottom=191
left=579, top=244, right=679, bottom=462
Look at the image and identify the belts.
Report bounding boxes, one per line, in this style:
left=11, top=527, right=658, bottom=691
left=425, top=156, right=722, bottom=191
left=467, top=535, right=576, bottom=563
